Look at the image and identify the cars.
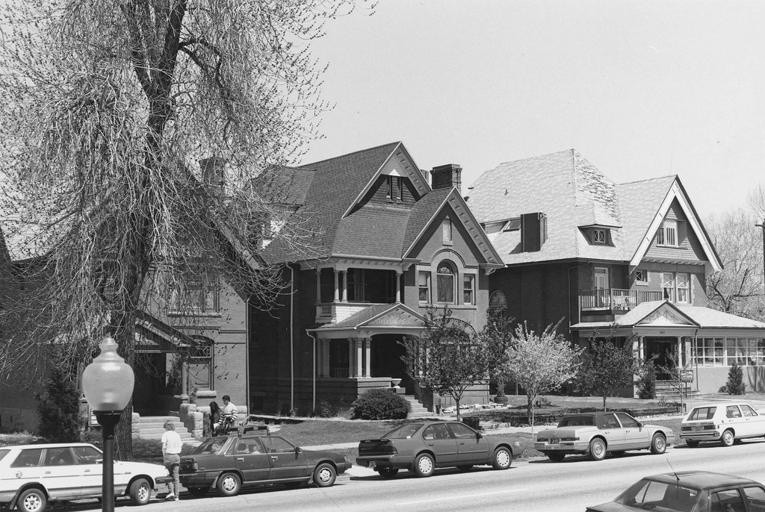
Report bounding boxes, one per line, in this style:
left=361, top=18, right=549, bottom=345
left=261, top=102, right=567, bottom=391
left=534, top=410, right=675, bottom=461
left=584, top=470, right=765, bottom=512
left=1, top=442, right=172, bottom=512
left=177, top=434, right=352, bottom=495
left=356, top=420, right=527, bottom=478
left=678, top=402, right=765, bottom=446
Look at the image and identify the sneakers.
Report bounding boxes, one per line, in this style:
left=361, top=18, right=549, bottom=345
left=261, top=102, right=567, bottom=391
left=165, top=493, right=179, bottom=501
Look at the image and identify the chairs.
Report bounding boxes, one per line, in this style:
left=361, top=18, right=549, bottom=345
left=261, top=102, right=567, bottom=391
left=237, top=442, right=259, bottom=453
left=660, top=486, right=690, bottom=509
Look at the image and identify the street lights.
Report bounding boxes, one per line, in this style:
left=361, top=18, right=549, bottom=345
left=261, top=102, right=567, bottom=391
left=80, top=333, right=135, bottom=512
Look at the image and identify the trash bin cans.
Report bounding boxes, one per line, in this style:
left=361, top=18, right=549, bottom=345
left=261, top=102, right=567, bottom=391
left=463, top=417, right=479, bottom=431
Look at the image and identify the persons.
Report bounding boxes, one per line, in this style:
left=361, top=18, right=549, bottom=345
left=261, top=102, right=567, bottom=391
left=208, top=395, right=238, bottom=438
left=161, top=421, right=182, bottom=501
